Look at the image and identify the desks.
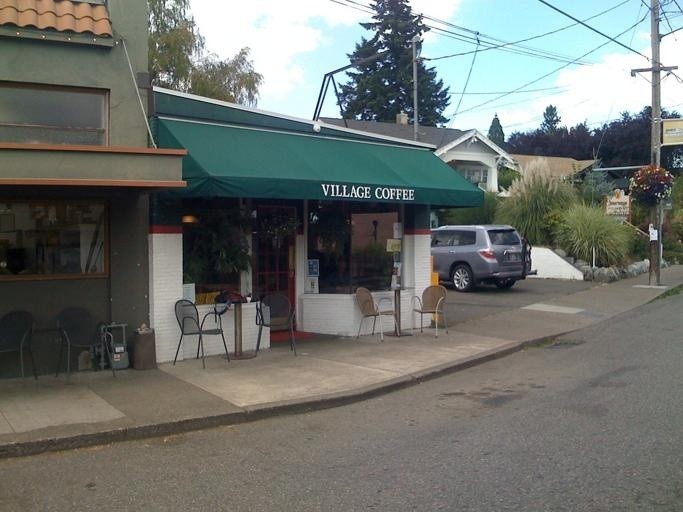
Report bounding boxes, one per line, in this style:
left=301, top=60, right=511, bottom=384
left=373, top=286, right=414, bottom=338
left=211, top=295, right=262, bottom=361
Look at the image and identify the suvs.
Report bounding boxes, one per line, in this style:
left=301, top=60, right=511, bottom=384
left=430, top=222, right=536, bottom=293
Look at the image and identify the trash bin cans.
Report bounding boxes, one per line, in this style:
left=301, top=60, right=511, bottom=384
left=132, top=323, right=155, bottom=370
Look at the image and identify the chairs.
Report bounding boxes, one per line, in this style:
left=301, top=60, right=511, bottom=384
left=252, top=293, right=298, bottom=357
left=53, top=305, right=117, bottom=385
left=406, top=284, right=449, bottom=338
left=355, top=286, right=400, bottom=344
left=171, top=298, right=229, bottom=370
left=0, top=308, right=39, bottom=382
